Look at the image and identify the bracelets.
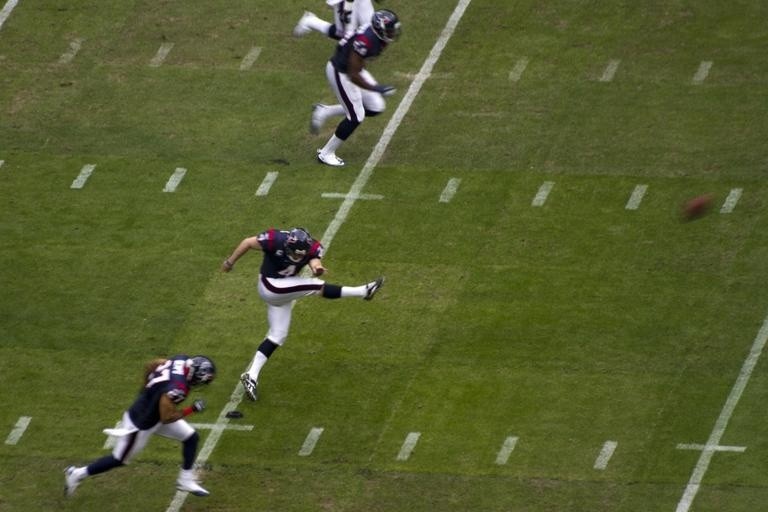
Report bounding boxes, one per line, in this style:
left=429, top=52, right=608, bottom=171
left=182, top=405, right=194, bottom=417
left=225, top=259, right=234, bottom=267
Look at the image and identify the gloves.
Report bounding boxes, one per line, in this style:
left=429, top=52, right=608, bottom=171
left=192, top=400, right=206, bottom=412
left=374, top=84, right=397, bottom=97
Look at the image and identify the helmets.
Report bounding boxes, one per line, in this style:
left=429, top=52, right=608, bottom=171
left=371, top=9, right=401, bottom=43
left=184, top=355, right=215, bottom=390
left=284, top=228, right=312, bottom=263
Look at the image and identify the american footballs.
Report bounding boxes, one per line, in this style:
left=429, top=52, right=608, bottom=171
left=683, top=196, right=717, bottom=220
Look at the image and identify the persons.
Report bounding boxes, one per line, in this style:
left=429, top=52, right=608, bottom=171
left=309, top=8, right=404, bottom=167
left=221, top=227, right=387, bottom=404
left=292, top=0, right=385, bottom=44
left=62, top=353, right=215, bottom=500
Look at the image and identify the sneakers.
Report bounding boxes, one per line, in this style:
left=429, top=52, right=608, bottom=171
left=240, top=372, right=257, bottom=401
left=176, top=479, right=210, bottom=496
left=312, top=103, right=326, bottom=135
left=316, top=149, right=345, bottom=166
left=292, top=12, right=316, bottom=36
left=63, top=465, right=81, bottom=499
left=363, top=277, right=385, bottom=300
